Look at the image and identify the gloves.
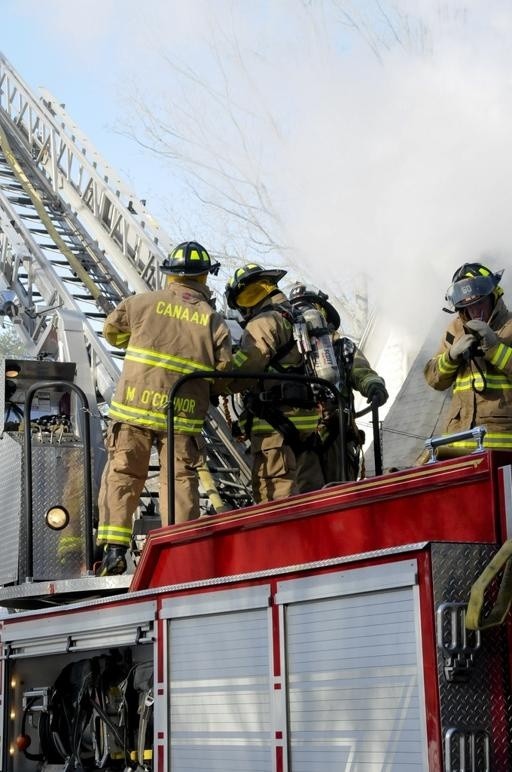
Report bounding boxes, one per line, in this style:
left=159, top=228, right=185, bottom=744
left=466, top=319, right=499, bottom=352
left=209, top=390, right=220, bottom=407
left=367, top=383, right=388, bottom=404
left=449, top=334, right=476, bottom=361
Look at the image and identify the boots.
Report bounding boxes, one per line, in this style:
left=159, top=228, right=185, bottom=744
left=95, top=543, right=128, bottom=576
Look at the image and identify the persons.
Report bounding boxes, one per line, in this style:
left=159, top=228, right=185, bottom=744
left=93, top=242, right=232, bottom=575
left=290, top=283, right=387, bottom=494
left=423, top=262, right=512, bottom=464
left=220, top=262, right=339, bottom=506
left=57, top=446, right=89, bottom=579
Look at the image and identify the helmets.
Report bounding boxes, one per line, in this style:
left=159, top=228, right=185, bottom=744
left=289, top=282, right=340, bottom=331
left=224, top=263, right=287, bottom=310
left=444, top=262, right=502, bottom=309
left=157, top=241, right=221, bottom=278
